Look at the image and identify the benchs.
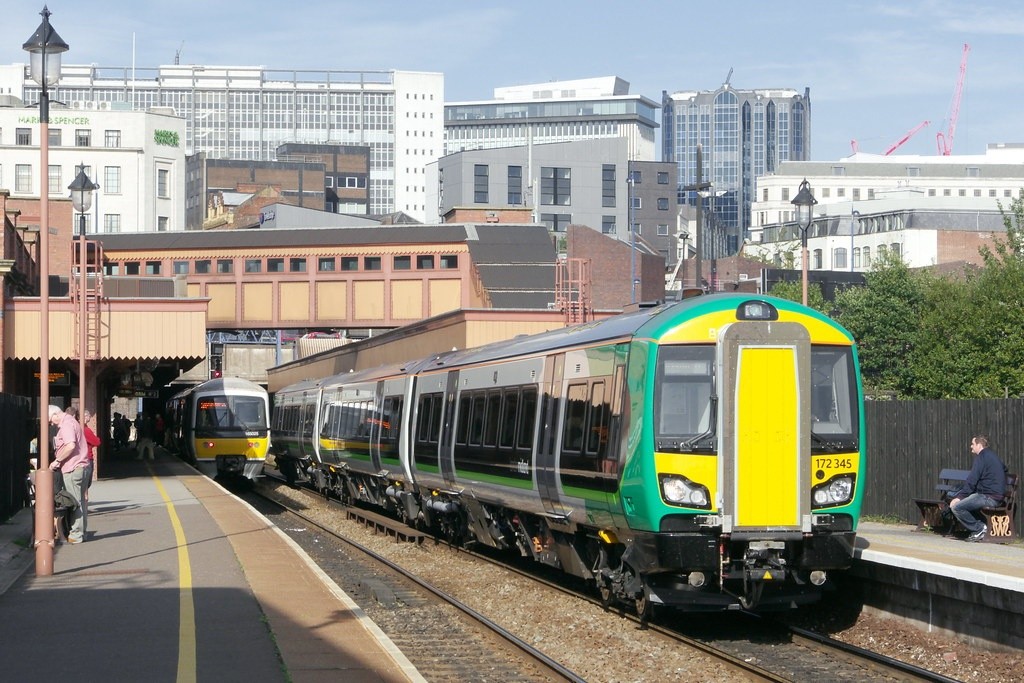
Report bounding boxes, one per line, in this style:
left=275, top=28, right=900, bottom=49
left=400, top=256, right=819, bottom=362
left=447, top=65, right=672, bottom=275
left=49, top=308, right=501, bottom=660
left=22, top=479, right=72, bottom=543
left=913, top=468, right=1019, bottom=544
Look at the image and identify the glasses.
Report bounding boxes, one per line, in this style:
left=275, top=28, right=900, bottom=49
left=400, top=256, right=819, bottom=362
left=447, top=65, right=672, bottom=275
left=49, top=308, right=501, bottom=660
left=48, top=413, right=56, bottom=425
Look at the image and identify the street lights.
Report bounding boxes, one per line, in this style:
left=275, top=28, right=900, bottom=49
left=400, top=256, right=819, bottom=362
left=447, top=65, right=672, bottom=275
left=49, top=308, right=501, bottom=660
left=66, top=159, right=100, bottom=439
left=696, top=186, right=728, bottom=294
left=790, top=177, right=819, bottom=307
left=22, top=3, right=70, bottom=578
left=672, top=231, right=689, bottom=300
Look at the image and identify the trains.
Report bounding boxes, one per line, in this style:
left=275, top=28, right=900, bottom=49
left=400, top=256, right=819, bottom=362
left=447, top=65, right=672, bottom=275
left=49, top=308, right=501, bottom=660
left=159, top=376, right=273, bottom=489
left=270, top=291, right=869, bottom=631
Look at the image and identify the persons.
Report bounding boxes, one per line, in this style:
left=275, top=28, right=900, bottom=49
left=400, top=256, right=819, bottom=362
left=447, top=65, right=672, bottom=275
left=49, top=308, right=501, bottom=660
left=112, top=412, right=132, bottom=452
left=30, top=404, right=101, bottom=544
left=133, top=413, right=164, bottom=464
left=949, top=435, right=1009, bottom=542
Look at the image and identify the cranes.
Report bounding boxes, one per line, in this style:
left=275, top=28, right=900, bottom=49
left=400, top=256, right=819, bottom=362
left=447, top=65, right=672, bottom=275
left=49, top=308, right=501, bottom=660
left=933, top=41, right=971, bottom=157
left=850, top=119, right=932, bottom=156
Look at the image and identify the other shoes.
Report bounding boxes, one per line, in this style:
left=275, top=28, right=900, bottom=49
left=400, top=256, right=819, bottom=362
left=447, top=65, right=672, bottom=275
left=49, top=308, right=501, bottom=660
left=966, top=523, right=987, bottom=542
left=65, top=536, right=86, bottom=543
